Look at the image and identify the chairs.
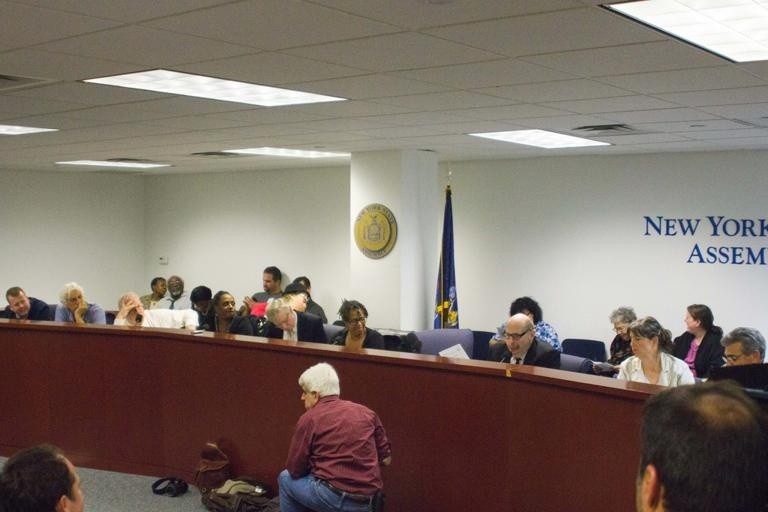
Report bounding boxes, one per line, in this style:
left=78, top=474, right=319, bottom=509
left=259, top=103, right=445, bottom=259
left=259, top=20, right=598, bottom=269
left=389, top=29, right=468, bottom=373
left=106, top=311, right=117, bottom=325
left=415, top=328, right=474, bottom=360
left=321, top=324, right=345, bottom=341
left=47, top=305, right=59, bottom=321
left=472, top=332, right=496, bottom=359
left=558, top=338, right=605, bottom=374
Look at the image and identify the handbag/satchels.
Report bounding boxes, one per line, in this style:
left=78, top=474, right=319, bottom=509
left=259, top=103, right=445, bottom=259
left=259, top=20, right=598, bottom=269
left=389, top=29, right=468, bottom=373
left=196, top=442, right=229, bottom=494
left=201, top=477, right=269, bottom=512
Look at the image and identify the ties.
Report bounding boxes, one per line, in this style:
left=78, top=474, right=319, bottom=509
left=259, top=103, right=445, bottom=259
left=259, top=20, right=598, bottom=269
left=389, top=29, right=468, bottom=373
left=169, top=301, right=174, bottom=310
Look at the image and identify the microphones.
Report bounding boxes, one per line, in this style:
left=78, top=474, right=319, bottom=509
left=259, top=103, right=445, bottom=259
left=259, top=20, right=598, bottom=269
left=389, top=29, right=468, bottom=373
left=499, top=350, right=512, bottom=363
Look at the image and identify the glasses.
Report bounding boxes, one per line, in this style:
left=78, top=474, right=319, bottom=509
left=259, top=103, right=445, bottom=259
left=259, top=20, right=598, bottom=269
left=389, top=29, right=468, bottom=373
left=502, top=329, right=529, bottom=340
left=346, top=316, right=364, bottom=326
left=722, top=352, right=742, bottom=362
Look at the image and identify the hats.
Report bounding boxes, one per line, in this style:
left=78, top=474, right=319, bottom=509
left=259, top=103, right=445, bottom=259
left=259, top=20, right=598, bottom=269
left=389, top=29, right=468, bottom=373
left=282, top=284, right=310, bottom=296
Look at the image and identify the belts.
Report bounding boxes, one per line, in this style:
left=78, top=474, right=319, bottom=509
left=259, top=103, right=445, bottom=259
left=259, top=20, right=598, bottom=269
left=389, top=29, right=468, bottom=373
left=321, top=481, right=369, bottom=505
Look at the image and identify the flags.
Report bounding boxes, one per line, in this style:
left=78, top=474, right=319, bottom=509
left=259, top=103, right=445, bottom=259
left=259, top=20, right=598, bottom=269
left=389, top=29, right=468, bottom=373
left=434, top=186, right=459, bottom=330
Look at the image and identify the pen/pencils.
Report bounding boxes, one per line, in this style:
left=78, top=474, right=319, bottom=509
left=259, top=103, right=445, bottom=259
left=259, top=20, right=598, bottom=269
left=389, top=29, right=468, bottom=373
left=191, top=331, right=204, bottom=334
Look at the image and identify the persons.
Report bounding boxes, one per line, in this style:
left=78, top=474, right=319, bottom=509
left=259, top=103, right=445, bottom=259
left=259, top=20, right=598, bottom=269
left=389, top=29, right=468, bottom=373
left=278, top=362, right=391, bottom=511
left=1, top=443, right=83, bottom=512
left=492, top=298, right=767, bottom=387
left=636, top=380, right=768, bottom=512
